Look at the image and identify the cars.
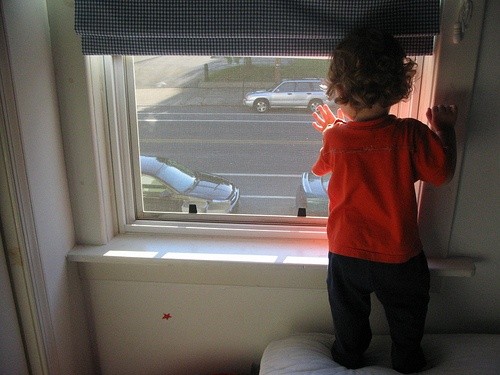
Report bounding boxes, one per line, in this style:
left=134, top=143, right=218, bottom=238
left=240, top=79, right=331, bottom=115
left=296, top=171, right=332, bottom=217
left=140, top=155, right=241, bottom=214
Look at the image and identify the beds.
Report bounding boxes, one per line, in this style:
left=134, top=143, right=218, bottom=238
left=257, top=331, right=500, bottom=375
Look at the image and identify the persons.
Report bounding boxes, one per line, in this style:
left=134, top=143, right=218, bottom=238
left=310, top=23, right=458, bottom=375
